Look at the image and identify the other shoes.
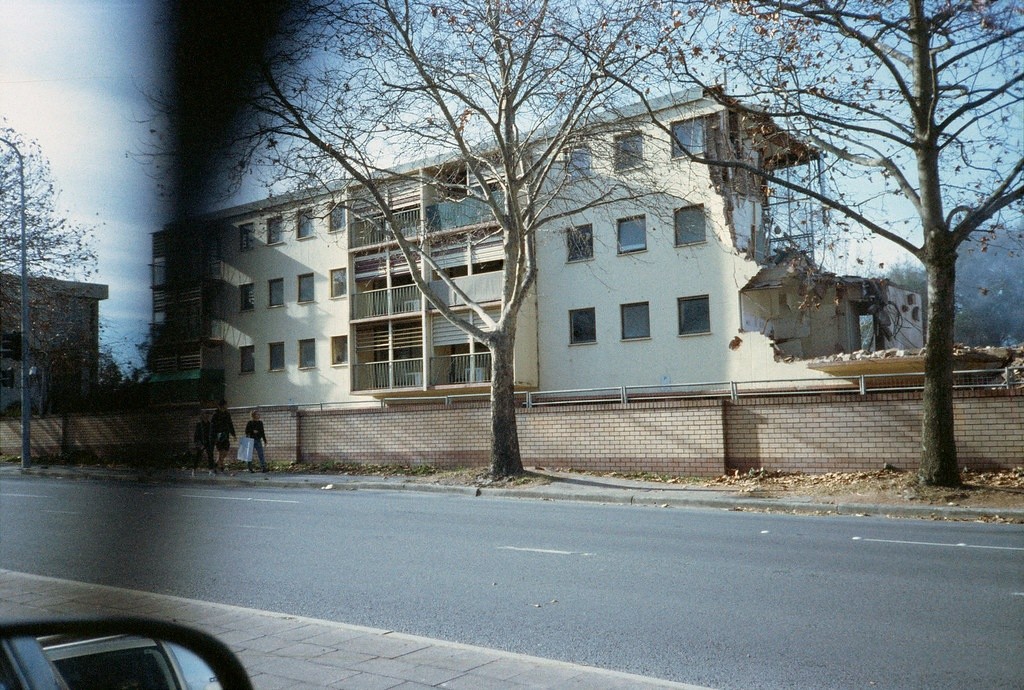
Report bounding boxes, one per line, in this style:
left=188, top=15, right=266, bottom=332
left=261, top=469, right=269, bottom=473
left=192, top=470, right=197, bottom=476
left=220, top=469, right=228, bottom=473
left=247, top=469, right=254, bottom=474
left=209, top=471, right=216, bottom=477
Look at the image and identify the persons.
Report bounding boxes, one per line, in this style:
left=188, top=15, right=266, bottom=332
left=191, top=411, right=216, bottom=478
left=243, top=411, right=269, bottom=473
left=210, top=399, right=238, bottom=475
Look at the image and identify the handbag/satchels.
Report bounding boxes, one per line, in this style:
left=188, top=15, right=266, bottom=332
left=214, top=432, right=227, bottom=442
left=236, top=434, right=255, bottom=462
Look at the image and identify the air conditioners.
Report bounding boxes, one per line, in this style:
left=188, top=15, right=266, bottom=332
left=404, top=299, right=421, bottom=314
left=400, top=227, right=419, bottom=239
left=405, top=370, right=423, bottom=387
left=464, top=365, right=489, bottom=382
